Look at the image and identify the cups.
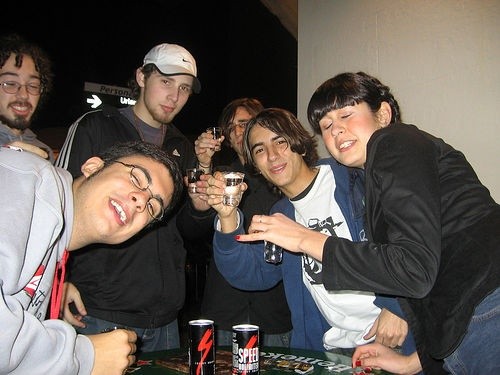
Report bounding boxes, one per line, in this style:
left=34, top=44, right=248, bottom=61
left=187, top=169, right=205, bottom=194
left=206, top=126, right=222, bottom=152
left=101, top=326, right=129, bottom=333
left=264, top=240, right=283, bottom=263
left=222, top=171, right=244, bottom=207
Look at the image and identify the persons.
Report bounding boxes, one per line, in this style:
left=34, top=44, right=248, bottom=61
left=0, top=32, right=54, bottom=163
left=53, top=44, right=211, bottom=354
left=0, top=138, right=184, bottom=375
left=206, top=108, right=425, bottom=375
left=195, top=98, right=294, bottom=347
left=234, top=72, right=500, bottom=375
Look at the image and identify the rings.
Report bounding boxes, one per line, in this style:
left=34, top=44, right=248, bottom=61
left=259, top=215, right=263, bottom=223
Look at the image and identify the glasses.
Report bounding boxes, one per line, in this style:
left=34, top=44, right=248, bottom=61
left=104, top=159, right=164, bottom=223
left=0, top=80, right=44, bottom=95
left=226, top=119, right=250, bottom=135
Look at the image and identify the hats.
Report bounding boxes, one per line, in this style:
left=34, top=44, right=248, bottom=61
left=143, top=43, right=201, bottom=94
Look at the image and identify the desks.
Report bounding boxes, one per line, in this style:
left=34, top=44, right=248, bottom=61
left=124, top=345, right=392, bottom=375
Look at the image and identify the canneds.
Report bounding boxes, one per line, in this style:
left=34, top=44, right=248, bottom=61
left=231, top=324, right=260, bottom=375
left=188, top=319, right=216, bottom=375
